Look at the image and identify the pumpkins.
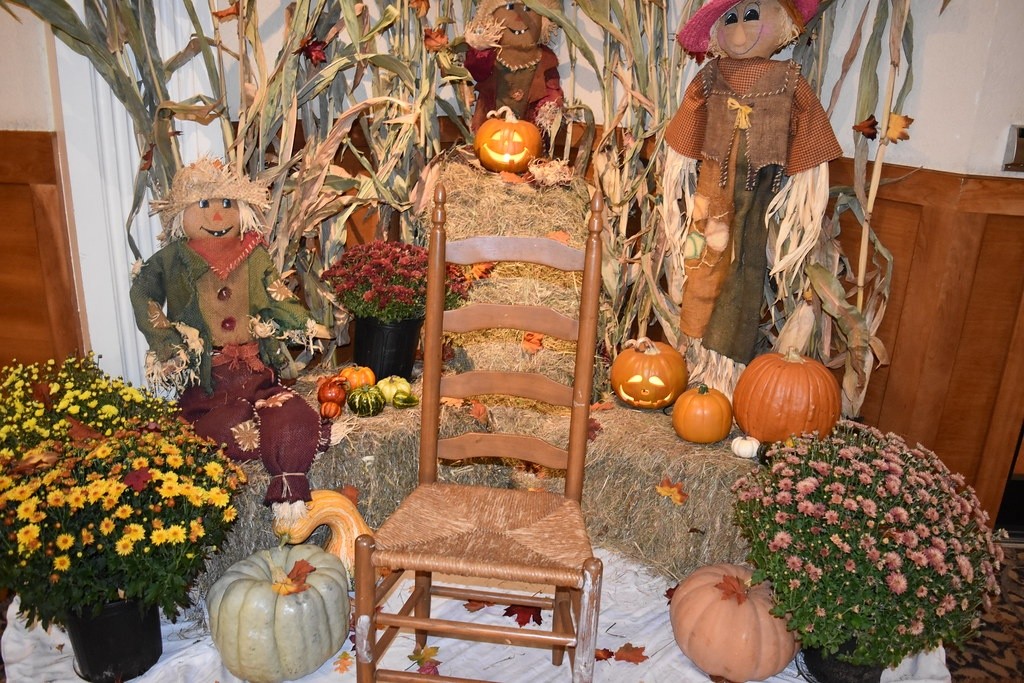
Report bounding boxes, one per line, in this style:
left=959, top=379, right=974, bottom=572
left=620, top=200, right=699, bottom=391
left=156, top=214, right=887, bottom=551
left=672, top=346, right=841, bottom=457
left=317, top=363, right=419, bottom=419
left=204, top=489, right=377, bottom=681
left=669, top=565, right=803, bottom=682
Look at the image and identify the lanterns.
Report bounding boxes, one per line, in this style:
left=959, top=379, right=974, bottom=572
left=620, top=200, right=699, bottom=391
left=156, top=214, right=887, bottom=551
left=609, top=337, right=689, bottom=408
left=473, top=105, right=543, bottom=173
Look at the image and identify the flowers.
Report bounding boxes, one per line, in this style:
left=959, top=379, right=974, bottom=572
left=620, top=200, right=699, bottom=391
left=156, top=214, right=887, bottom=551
left=0, top=349, right=248, bottom=634
left=731, top=417, right=1010, bottom=672
left=319, top=239, right=473, bottom=324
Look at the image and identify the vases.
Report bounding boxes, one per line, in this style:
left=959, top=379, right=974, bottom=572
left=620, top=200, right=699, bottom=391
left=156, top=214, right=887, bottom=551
left=60, top=595, right=164, bottom=683
left=354, top=318, right=424, bottom=384
left=800, top=635, right=886, bottom=683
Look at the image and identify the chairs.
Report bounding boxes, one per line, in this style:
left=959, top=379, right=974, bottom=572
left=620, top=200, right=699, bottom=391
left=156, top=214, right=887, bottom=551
left=354, top=184, right=603, bottom=683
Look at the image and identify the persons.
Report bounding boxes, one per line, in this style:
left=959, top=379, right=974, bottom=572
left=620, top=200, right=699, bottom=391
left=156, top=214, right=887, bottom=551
left=128, top=156, right=332, bottom=518
left=461, top=0, right=568, bottom=144
left=652, top=0, right=841, bottom=390
